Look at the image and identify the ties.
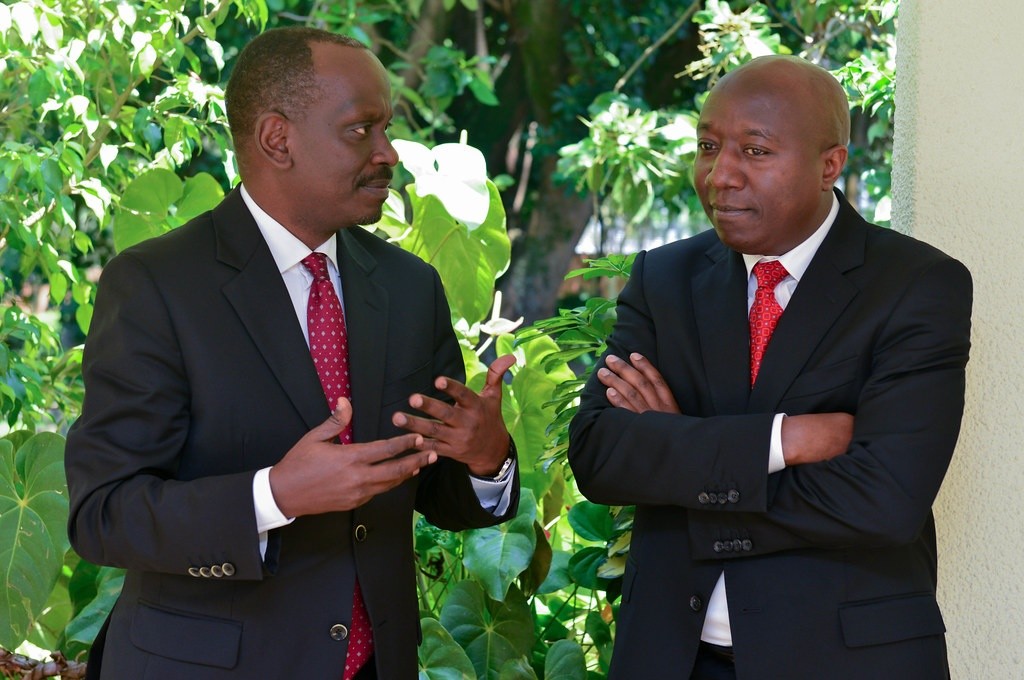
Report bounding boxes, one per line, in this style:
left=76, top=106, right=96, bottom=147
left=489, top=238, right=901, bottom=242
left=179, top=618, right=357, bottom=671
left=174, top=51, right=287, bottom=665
left=746, top=262, right=789, bottom=390
left=300, top=253, right=375, bottom=680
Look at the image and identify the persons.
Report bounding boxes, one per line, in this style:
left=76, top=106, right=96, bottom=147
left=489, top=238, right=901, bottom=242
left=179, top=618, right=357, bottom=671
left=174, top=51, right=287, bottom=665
left=566, top=53, right=975, bottom=680
left=64, top=25, right=520, bottom=680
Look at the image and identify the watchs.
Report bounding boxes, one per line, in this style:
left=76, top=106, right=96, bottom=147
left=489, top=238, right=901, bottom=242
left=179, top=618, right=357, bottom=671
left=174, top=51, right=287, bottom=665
left=485, top=442, right=514, bottom=485
